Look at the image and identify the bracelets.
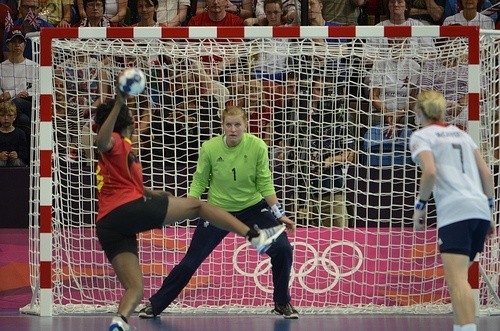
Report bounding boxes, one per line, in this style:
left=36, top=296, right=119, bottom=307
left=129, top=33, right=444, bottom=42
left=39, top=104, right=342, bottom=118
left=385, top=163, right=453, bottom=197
left=489, top=198, right=495, bottom=208
left=415, top=198, right=428, bottom=210
left=270, top=202, right=285, bottom=220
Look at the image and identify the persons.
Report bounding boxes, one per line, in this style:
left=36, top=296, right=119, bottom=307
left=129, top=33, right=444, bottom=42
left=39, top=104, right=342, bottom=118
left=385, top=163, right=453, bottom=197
left=0, top=0, right=357, bottom=227
left=410, top=90, right=495, bottom=331
left=95, top=66, right=286, bottom=331
left=362, top=0, right=500, bottom=225
left=138, top=106, right=300, bottom=319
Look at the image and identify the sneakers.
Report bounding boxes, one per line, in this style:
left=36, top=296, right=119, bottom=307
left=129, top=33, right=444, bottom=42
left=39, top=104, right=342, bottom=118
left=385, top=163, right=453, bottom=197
left=250, top=224, right=286, bottom=254
left=109, top=317, right=131, bottom=331
left=273, top=303, right=299, bottom=319
left=138, top=303, right=160, bottom=317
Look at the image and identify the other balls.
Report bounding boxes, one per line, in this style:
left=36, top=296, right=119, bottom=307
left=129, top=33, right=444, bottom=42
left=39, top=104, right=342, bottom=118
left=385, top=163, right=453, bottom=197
left=118, top=67, right=146, bottom=95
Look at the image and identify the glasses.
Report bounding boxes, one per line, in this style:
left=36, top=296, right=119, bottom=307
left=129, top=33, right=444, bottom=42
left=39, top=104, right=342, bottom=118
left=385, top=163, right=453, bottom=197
left=87, top=2, right=104, bottom=9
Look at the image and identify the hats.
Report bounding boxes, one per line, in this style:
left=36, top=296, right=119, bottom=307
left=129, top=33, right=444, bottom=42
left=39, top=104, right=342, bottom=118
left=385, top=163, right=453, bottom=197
left=6, top=29, right=27, bottom=43
